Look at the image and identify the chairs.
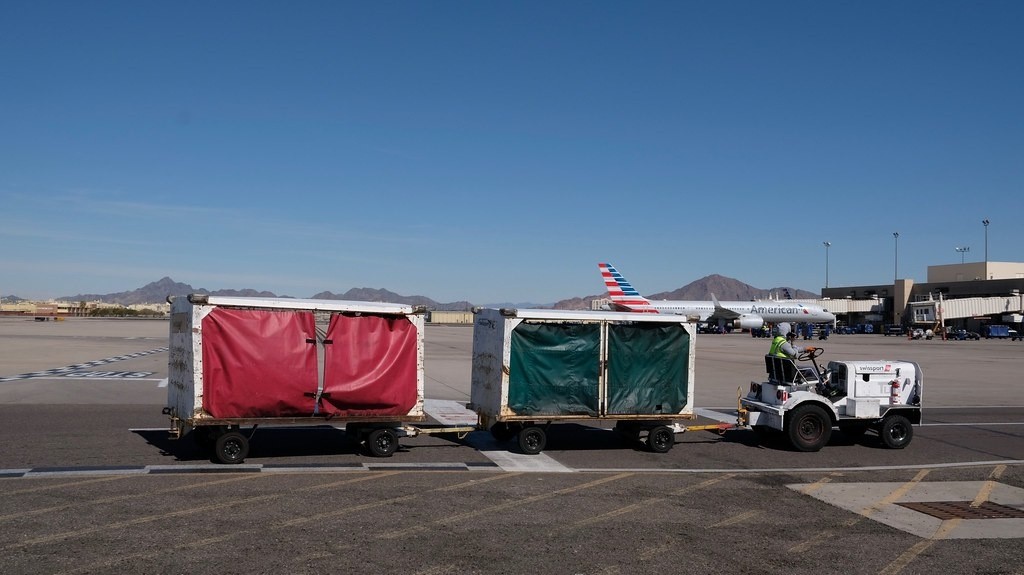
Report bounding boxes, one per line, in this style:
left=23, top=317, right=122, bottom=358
left=764, top=354, right=821, bottom=394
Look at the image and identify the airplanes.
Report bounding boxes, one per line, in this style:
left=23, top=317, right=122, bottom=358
left=893, top=231, right=899, bottom=281
left=599, top=262, right=836, bottom=340
left=982, top=218, right=989, bottom=279
left=823, top=241, right=831, bottom=288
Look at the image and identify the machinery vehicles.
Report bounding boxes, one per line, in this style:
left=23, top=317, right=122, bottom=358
left=156, top=282, right=923, bottom=451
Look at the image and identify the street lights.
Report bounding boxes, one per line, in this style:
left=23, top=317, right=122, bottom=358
left=955, top=247, right=970, bottom=264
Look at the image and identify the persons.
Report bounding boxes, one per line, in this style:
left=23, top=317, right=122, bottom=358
left=771, top=322, right=838, bottom=397
